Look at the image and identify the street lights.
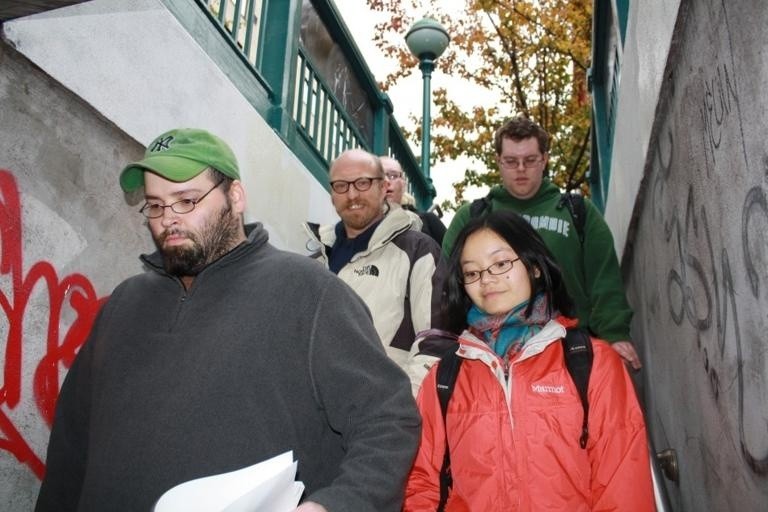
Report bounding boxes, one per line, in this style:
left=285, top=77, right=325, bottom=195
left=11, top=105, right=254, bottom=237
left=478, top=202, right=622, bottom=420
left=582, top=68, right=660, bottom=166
left=406, top=17, right=452, bottom=212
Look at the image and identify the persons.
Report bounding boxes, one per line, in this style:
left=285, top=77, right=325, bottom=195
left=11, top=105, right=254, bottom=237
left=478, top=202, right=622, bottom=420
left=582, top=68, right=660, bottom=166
left=397, top=207, right=660, bottom=511
left=301, top=147, right=444, bottom=402
left=28, top=130, right=426, bottom=511
left=378, top=153, right=449, bottom=247
left=440, top=120, right=643, bottom=371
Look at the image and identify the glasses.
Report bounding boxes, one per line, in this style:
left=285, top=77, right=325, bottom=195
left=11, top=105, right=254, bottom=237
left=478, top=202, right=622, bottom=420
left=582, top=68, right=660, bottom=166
left=329, top=177, right=382, bottom=193
left=385, top=170, right=403, bottom=179
left=499, top=156, right=543, bottom=168
left=458, top=257, right=519, bottom=285
left=140, top=180, right=224, bottom=219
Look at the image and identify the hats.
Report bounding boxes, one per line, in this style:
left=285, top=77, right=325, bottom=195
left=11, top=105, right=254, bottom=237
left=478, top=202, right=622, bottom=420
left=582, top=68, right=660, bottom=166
left=119, top=128, right=238, bottom=192
